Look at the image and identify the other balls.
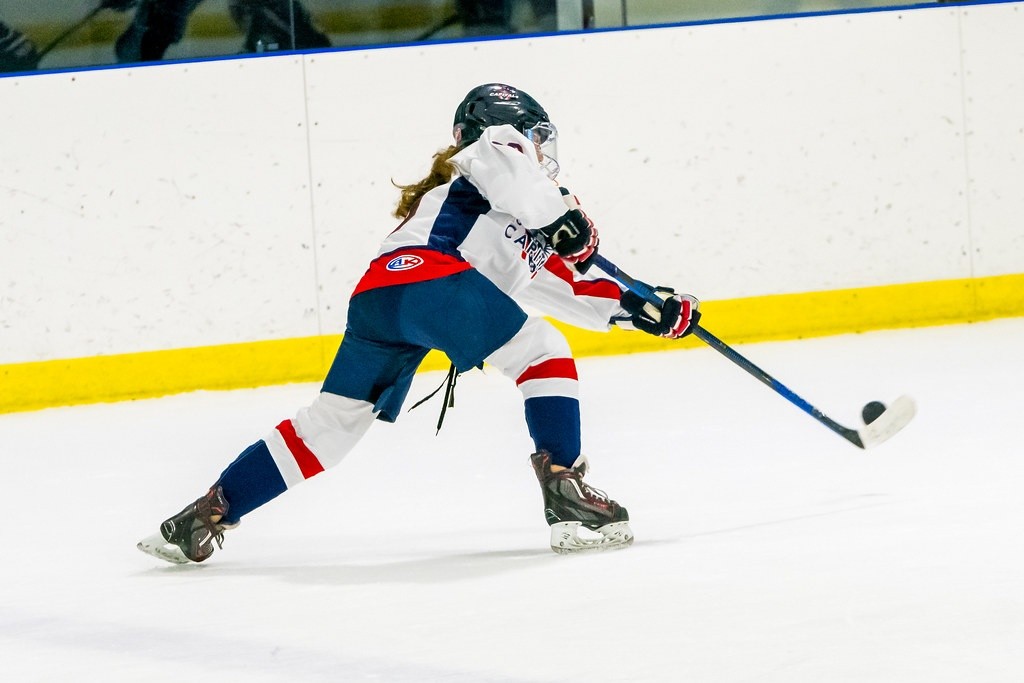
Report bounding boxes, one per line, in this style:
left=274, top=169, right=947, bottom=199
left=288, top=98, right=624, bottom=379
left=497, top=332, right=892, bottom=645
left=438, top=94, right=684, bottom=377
left=861, top=400, right=887, bottom=425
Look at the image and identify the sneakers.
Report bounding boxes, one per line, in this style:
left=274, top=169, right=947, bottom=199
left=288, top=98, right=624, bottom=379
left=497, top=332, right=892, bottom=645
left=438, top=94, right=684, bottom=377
left=530, top=451, right=634, bottom=550
left=137, top=486, right=241, bottom=565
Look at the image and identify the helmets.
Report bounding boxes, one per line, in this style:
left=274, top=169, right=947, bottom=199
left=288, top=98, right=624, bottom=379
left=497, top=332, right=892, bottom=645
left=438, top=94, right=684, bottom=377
left=453, top=83, right=552, bottom=146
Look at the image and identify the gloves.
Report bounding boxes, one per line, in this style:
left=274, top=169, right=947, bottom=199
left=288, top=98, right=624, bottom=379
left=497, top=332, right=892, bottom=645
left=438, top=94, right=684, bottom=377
left=533, top=209, right=599, bottom=265
left=607, top=281, right=702, bottom=340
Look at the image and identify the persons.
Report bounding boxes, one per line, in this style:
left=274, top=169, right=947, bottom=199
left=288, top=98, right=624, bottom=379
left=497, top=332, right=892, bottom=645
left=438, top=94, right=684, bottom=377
left=136, top=83, right=701, bottom=567
left=99, top=0, right=333, bottom=63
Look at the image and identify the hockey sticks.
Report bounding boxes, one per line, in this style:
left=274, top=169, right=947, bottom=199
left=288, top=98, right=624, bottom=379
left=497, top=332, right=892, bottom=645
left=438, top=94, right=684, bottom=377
left=589, top=253, right=921, bottom=451
